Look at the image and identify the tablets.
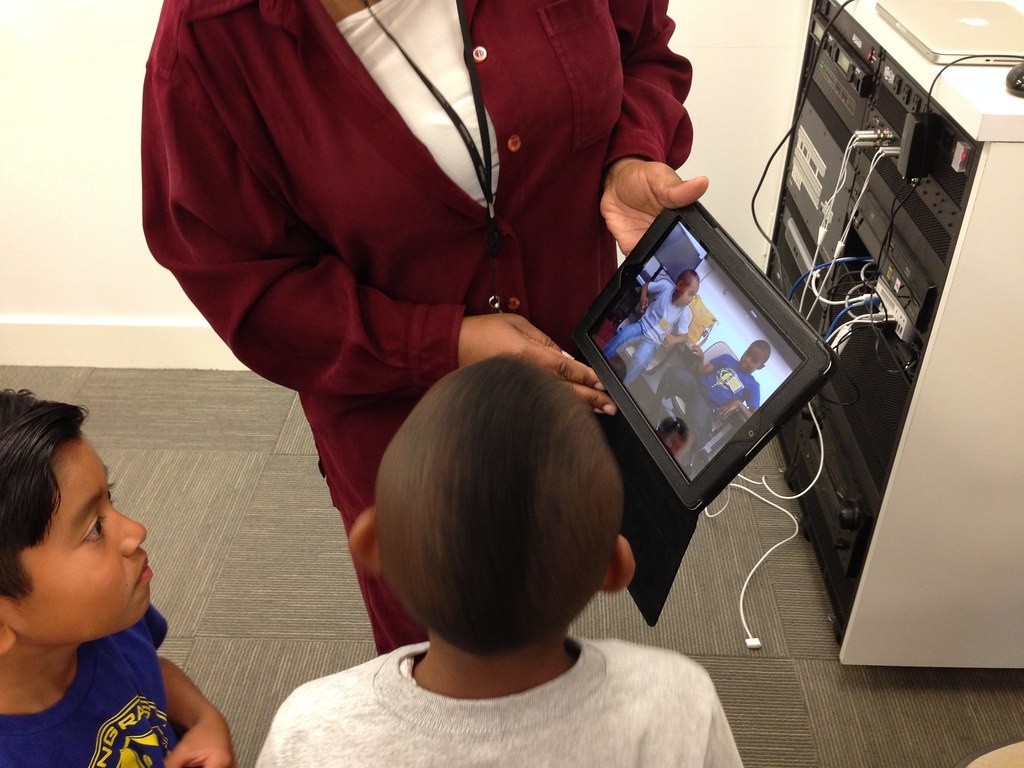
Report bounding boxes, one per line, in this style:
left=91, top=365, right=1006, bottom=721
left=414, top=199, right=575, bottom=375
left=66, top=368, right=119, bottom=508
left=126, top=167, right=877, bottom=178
left=571, top=200, right=838, bottom=514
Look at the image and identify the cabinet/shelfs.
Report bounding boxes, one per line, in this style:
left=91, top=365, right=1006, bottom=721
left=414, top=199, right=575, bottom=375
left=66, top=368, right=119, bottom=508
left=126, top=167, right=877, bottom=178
left=837, top=0, right=1022, bottom=670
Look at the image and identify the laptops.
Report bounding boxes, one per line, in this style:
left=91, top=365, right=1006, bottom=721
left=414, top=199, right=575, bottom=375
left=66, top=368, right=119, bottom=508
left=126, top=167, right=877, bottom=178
left=877, top=0, right=1024, bottom=65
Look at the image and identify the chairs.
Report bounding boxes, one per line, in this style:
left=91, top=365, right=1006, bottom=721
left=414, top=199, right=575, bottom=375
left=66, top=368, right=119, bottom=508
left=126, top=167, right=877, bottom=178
left=657, top=341, right=747, bottom=456
left=617, top=292, right=716, bottom=377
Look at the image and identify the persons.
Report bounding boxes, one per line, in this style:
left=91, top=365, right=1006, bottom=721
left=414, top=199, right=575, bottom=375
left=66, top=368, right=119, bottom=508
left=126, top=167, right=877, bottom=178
left=257, top=347, right=746, bottom=766
left=0, top=387, right=238, bottom=768
left=140, top=0, right=711, bottom=655
left=604, top=268, right=772, bottom=462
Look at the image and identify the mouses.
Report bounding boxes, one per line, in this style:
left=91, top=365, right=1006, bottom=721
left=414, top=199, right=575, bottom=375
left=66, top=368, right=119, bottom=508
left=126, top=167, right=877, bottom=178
left=1006, top=62, right=1024, bottom=97
